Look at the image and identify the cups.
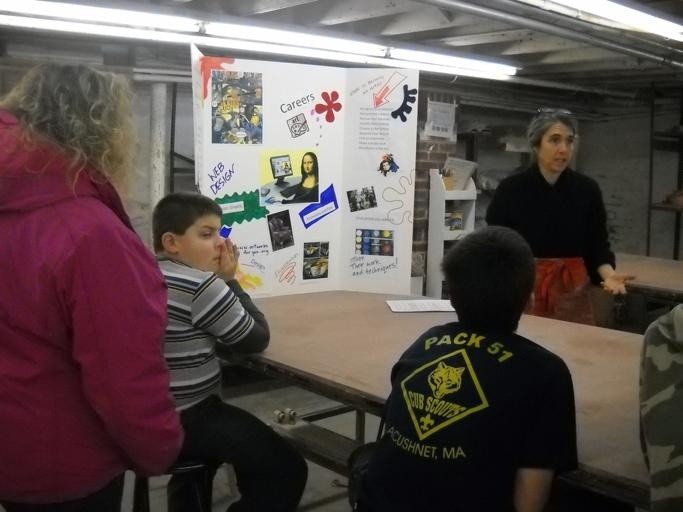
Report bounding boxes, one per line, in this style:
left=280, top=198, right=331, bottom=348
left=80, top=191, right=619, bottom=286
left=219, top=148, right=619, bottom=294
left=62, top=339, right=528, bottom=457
left=441, top=176, right=454, bottom=192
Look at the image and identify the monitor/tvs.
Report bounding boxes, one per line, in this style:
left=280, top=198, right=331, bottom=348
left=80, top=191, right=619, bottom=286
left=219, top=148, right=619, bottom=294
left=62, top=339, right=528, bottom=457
left=270, top=154, right=293, bottom=186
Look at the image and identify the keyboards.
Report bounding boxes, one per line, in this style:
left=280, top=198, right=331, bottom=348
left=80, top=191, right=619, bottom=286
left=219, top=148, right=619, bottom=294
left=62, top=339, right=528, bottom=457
left=386, top=299, right=456, bottom=312
left=280, top=182, right=299, bottom=198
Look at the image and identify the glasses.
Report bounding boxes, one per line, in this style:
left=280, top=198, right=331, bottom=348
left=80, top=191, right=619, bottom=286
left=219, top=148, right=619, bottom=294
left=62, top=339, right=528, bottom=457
left=535, top=104, right=573, bottom=120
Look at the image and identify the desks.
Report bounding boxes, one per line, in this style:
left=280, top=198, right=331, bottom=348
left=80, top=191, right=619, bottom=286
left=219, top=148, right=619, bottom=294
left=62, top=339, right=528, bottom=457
left=216, top=288, right=653, bottom=509
left=612, top=252, right=683, bottom=335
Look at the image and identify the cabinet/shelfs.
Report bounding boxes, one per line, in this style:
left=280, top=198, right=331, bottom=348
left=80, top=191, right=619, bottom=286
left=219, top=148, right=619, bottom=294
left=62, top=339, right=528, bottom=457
left=424, top=169, right=477, bottom=300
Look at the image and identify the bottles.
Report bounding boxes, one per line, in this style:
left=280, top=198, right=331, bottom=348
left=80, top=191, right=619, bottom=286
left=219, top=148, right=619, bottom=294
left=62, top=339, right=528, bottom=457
left=445, top=211, right=463, bottom=232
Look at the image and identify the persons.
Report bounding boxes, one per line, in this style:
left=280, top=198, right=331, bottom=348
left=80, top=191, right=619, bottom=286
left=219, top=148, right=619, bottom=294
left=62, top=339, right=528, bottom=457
left=269, top=152, right=319, bottom=204
left=347, top=226, right=580, bottom=512
left=485, top=108, right=636, bottom=326
left=639, top=303, right=682, bottom=512
left=0, top=60, right=185, bottom=512
left=153, top=193, right=308, bottom=512
